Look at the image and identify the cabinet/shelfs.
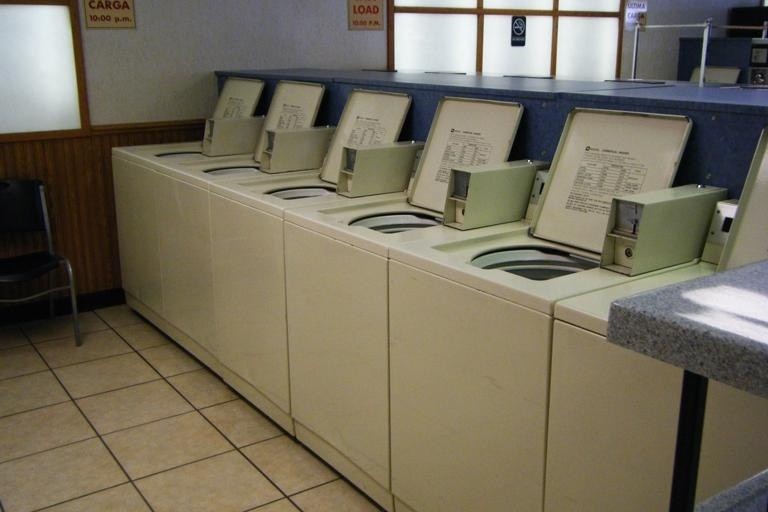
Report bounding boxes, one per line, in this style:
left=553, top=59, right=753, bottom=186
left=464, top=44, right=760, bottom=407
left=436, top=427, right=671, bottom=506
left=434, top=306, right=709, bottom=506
left=608, top=260, right=768, bottom=512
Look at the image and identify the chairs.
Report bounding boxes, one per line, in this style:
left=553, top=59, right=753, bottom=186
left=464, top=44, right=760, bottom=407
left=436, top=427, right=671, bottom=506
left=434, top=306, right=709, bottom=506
left=0, top=177, right=81, bottom=346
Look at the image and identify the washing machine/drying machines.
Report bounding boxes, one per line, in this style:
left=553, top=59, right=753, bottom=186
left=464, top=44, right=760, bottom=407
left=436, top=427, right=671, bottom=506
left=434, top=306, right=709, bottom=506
left=109, top=69, right=767, bottom=512
